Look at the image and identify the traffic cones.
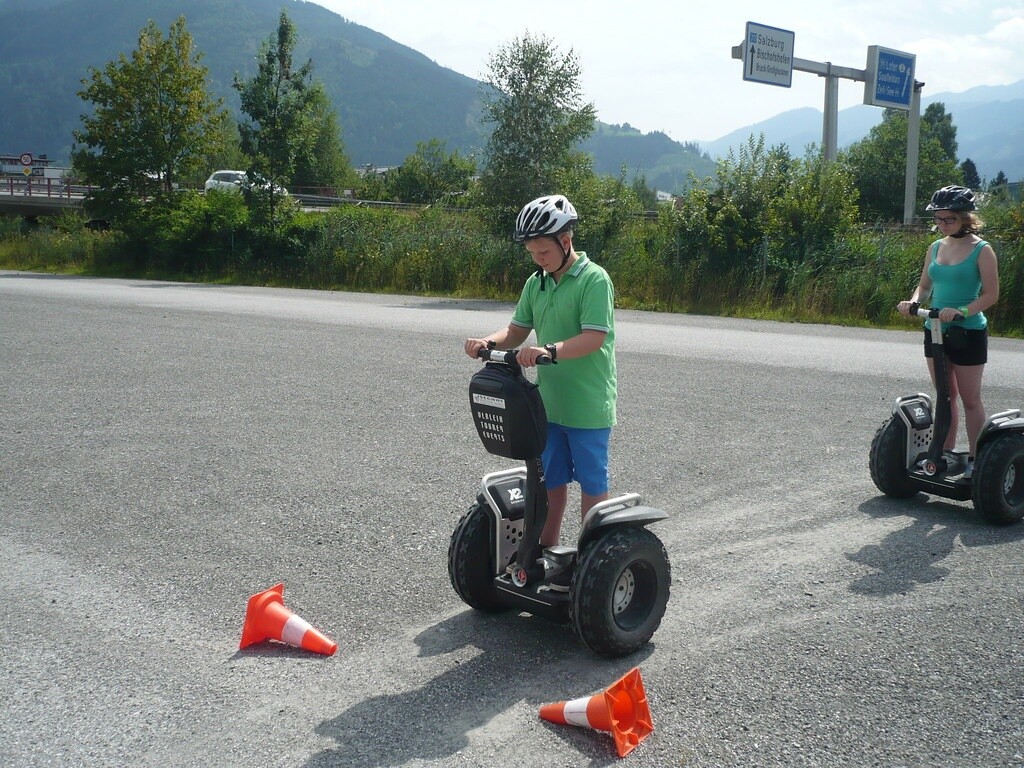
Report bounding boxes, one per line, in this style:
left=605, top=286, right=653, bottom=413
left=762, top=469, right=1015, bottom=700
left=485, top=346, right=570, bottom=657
left=538, top=666, right=653, bottom=759
left=238, top=585, right=337, bottom=657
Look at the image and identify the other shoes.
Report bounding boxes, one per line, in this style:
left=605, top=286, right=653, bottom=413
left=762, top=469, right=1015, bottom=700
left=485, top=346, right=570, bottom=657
left=917, top=459, right=929, bottom=468
left=550, top=552, right=577, bottom=593
left=506, top=562, right=517, bottom=575
left=964, top=460, right=974, bottom=479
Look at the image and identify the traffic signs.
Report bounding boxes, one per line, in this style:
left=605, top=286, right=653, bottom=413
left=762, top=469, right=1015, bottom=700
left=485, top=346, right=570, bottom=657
left=741, top=21, right=796, bottom=89
left=861, top=44, right=918, bottom=111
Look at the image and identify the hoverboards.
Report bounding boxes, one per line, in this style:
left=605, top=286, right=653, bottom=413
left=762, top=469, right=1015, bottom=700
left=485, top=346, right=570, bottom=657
left=866, top=301, right=1024, bottom=527
left=444, top=341, right=675, bottom=660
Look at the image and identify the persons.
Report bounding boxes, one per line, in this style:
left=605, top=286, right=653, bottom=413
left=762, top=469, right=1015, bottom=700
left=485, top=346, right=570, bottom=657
left=464, top=194, right=617, bottom=594
left=896, top=185, right=1000, bottom=480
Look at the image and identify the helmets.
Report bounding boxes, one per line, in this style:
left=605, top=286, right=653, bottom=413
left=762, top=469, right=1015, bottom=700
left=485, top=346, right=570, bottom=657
left=514, top=194, right=578, bottom=242
left=925, top=185, right=979, bottom=211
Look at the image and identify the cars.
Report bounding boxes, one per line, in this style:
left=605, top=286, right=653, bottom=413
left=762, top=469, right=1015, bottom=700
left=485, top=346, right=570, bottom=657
left=204, top=168, right=288, bottom=198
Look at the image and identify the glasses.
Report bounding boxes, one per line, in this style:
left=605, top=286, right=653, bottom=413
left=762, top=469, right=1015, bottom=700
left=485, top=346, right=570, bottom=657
left=933, top=215, right=965, bottom=225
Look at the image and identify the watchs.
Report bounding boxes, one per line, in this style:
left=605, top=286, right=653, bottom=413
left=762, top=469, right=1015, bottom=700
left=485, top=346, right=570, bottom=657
left=958, top=306, right=968, bottom=318
left=544, top=342, right=557, bottom=362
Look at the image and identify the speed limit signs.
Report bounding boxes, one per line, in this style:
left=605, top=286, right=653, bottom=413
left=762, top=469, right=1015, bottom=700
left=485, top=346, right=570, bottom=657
left=21, top=154, right=32, bottom=166
